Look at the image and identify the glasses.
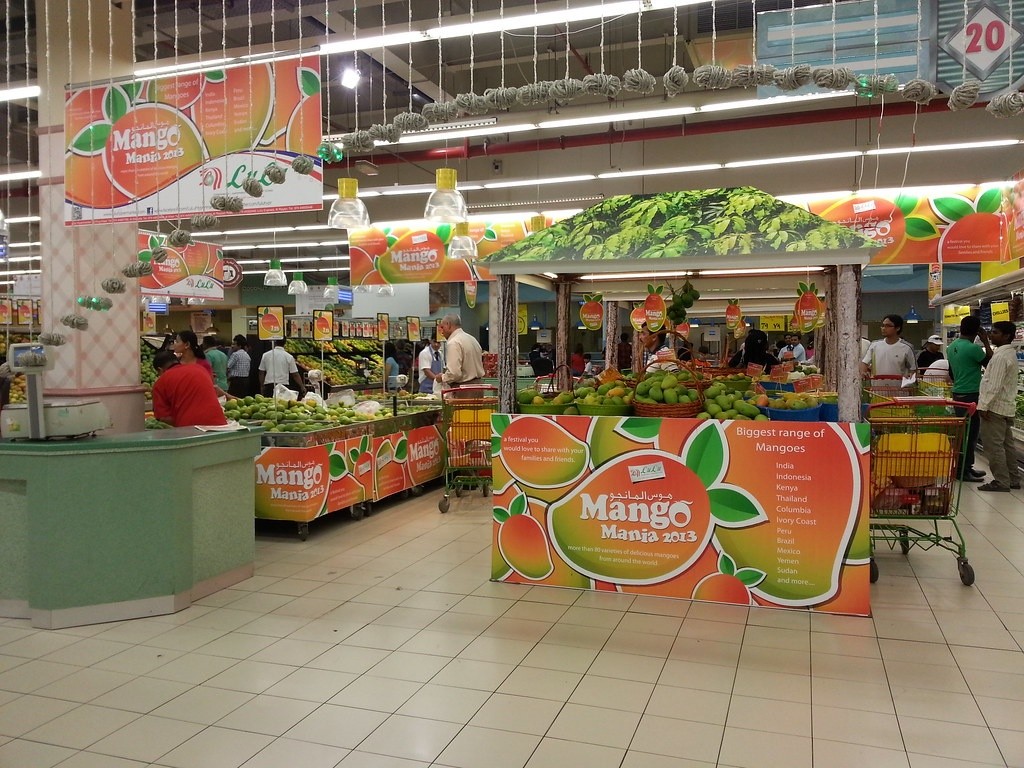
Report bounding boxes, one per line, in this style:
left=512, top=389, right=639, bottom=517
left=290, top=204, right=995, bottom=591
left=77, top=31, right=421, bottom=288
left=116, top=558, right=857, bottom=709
left=881, top=324, right=894, bottom=329
left=933, top=337, right=942, bottom=341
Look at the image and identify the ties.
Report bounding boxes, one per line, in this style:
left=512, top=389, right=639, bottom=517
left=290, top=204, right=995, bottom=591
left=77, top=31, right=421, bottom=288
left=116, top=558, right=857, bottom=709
left=435, top=352, right=439, bottom=361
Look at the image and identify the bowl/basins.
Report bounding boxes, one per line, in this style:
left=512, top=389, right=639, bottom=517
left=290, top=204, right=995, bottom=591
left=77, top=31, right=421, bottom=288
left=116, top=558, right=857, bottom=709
left=756, top=381, right=870, bottom=421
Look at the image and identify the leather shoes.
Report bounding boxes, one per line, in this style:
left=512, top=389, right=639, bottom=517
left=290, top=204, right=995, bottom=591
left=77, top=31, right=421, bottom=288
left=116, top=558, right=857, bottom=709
left=970, top=469, right=986, bottom=477
left=957, top=468, right=984, bottom=482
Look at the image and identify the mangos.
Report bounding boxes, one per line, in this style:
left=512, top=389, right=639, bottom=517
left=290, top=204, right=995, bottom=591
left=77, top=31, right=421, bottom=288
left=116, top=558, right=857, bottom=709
left=666, top=283, right=700, bottom=324
left=9, top=374, right=28, bottom=403
left=0, top=334, right=39, bottom=356
left=515, top=365, right=838, bottom=420
left=138, top=338, right=439, bottom=433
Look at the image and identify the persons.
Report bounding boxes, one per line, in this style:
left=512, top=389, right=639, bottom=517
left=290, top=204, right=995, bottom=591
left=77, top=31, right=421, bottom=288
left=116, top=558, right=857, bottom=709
left=945, top=316, right=994, bottom=482
left=436, top=313, right=486, bottom=399
left=202, top=336, right=229, bottom=393
left=397, top=336, right=429, bottom=393
left=226, top=333, right=307, bottom=400
left=151, top=351, right=227, bottom=427
left=617, top=332, right=632, bottom=373
left=0, top=355, right=15, bottom=414
left=417, top=333, right=445, bottom=394
left=384, top=342, right=399, bottom=383
left=676, top=342, right=693, bottom=361
left=638, top=321, right=680, bottom=374
left=173, top=331, right=240, bottom=401
left=726, top=329, right=815, bottom=375
left=976, top=321, right=1022, bottom=493
left=528, top=343, right=594, bottom=377
left=916, top=334, right=945, bottom=377
left=861, top=313, right=919, bottom=396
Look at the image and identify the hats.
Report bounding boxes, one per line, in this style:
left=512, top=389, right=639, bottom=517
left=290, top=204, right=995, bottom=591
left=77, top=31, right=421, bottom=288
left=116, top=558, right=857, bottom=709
left=927, top=335, right=944, bottom=344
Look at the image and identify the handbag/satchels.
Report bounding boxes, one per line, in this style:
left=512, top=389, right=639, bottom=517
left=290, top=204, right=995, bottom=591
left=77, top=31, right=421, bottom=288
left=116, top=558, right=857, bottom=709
left=736, top=348, right=746, bottom=369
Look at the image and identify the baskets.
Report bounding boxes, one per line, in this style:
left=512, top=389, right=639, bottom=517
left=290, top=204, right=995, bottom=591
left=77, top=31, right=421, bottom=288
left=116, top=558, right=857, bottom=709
left=517, top=331, right=747, bottom=419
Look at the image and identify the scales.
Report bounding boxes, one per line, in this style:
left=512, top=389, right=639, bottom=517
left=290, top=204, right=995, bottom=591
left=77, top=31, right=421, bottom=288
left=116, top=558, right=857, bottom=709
left=516, top=352, right=536, bottom=378
left=389, top=375, right=409, bottom=416
left=1, top=342, right=114, bottom=439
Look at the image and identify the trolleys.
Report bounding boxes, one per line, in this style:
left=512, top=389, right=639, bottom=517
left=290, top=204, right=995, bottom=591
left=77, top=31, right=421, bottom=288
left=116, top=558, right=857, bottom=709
left=866, top=367, right=977, bottom=587
left=438, top=383, right=498, bottom=514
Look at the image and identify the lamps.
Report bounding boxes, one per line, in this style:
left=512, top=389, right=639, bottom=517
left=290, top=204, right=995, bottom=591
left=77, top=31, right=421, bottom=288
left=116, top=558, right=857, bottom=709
left=280, top=256, right=320, bottom=262
left=319, top=238, right=349, bottom=247
left=134, top=0, right=1024, bottom=200
left=236, top=254, right=266, bottom=264
left=325, top=177, right=370, bottom=230
left=321, top=255, right=350, bottom=261
left=322, top=260, right=340, bottom=298
left=264, top=215, right=287, bottom=286
left=256, top=240, right=319, bottom=249
left=0, top=240, right=41, bottom=284
left=353, top=284, right=371, bottom=294
left=376, top=284, right=394, bottom=297
left=141, top=267, right=268, bottom=305
left=288, top=246, right=309, bottom=295
left=282, top=268, right=319, bottom=272
left=423, top=139, right=467, bottom=224
left=318, top=267, right=350, bottom=272
left=222, top=237, right=256, bottom=251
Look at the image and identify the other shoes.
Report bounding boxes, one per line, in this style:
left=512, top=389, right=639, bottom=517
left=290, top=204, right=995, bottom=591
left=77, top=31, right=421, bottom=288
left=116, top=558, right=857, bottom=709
left=978, top=480, right=1010, bottom=492
left=1010, top=485, right=1021, bottom=489
